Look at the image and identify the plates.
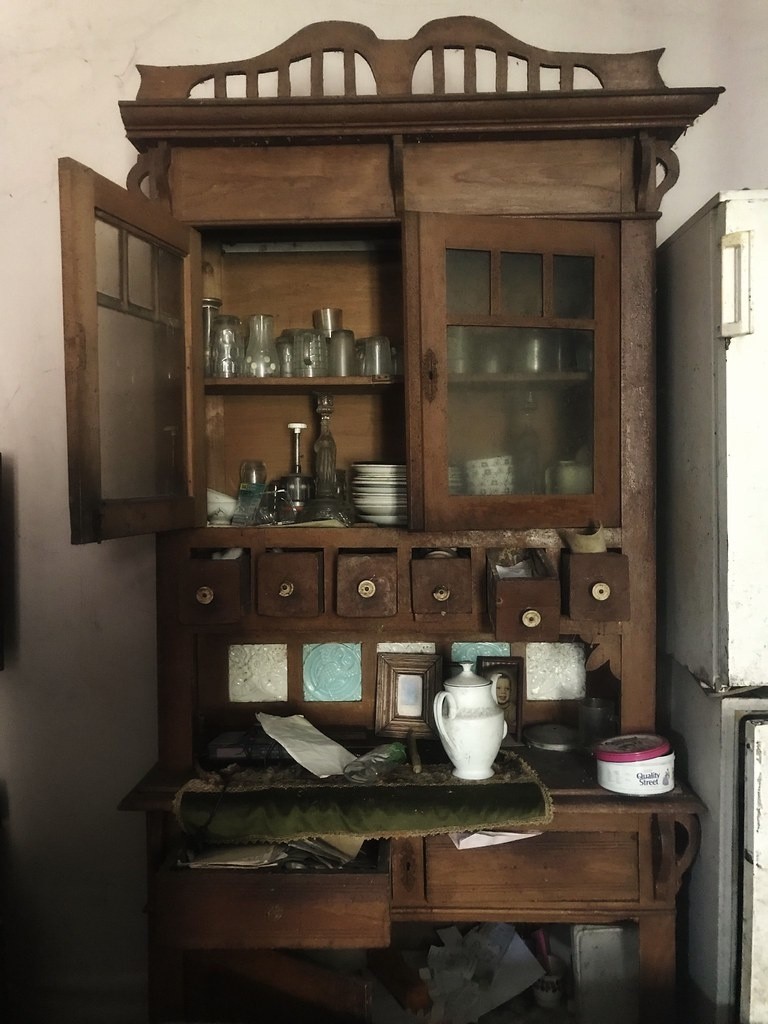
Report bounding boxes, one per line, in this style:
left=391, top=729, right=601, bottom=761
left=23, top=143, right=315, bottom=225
left=350, top=463, right=409, bottom=525
left=448, top=466, right=464, bottom=494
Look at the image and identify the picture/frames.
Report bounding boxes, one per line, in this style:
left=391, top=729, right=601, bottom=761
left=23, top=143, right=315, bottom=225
left=376, top=653, right=441, bottom=743
left=476, top=654, right=524, bottom=750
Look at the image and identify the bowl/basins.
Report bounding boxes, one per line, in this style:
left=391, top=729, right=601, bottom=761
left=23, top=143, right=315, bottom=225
left=594, top=732, right=675, bottom=797
left=466, top=455, right=514, bottom=497
left=207, top=488, right=239, bottom=527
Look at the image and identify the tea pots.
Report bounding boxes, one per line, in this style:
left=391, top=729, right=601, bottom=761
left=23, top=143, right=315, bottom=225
left=433, top=660, right=508, bottom=780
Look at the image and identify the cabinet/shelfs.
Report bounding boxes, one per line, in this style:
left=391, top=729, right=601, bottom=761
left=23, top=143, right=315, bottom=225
left=652, top=658, right=767, bottom=1024
left=659, top=184, right=766, bottom=702
left=54, top=12, right=729, bottom=1023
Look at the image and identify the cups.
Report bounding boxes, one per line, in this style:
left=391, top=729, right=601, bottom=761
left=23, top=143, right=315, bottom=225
left=445, top=324, right=594, bottom=375
left=204, top=306, right=408, bottom=378
left=534, top=974, right=563, bottom=1008
left=558, top=460, right=594, bottom=494
left=578, top=697, right=620, bottom=749
